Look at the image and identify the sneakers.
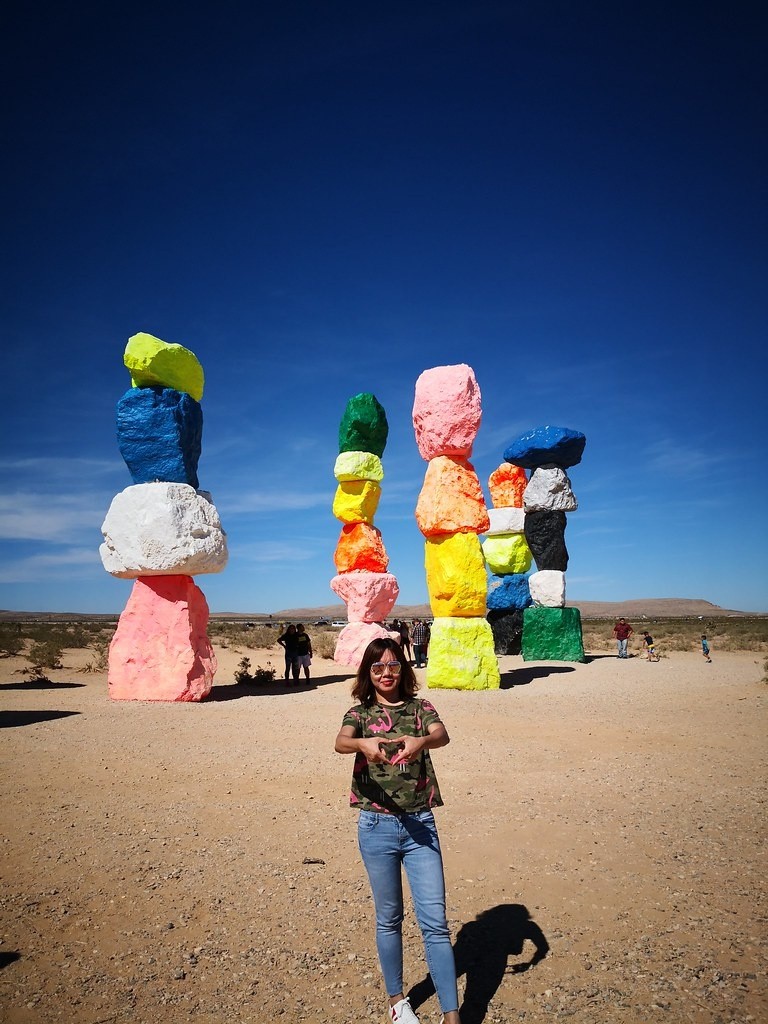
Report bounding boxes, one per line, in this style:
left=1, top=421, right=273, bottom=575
left=389, top=996, right=420, bottom=1024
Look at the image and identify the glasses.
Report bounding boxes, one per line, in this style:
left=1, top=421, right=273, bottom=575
left=371, top=661, right=402, bottom=675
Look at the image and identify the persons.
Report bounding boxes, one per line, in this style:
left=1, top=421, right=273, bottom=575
left=614, top=618, right=633, bottom=659
left=390, top=618, right=431, bottom=668
left=277, top=624, right=312, bottom=685
left=335, top=637, right=462, bottom=1024
left=642, top=631, right=660, bottom=662
left=701, top=635, right=712, bottom=663
